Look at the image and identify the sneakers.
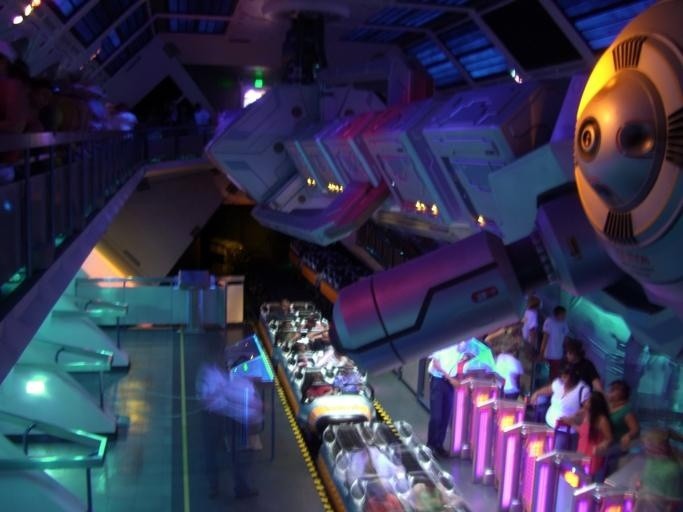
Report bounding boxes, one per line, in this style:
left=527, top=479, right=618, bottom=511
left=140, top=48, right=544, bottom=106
left=429, top=445, right=452, bottom=458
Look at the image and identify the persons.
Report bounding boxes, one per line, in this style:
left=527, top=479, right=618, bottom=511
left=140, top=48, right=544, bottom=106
left=427, top=294, right=681, bottom=511
left=223, top=350, right=273, bottom=501
left=191, top=334, right=231, bottom=498
left=2, top=51, right=210, bottom=282
